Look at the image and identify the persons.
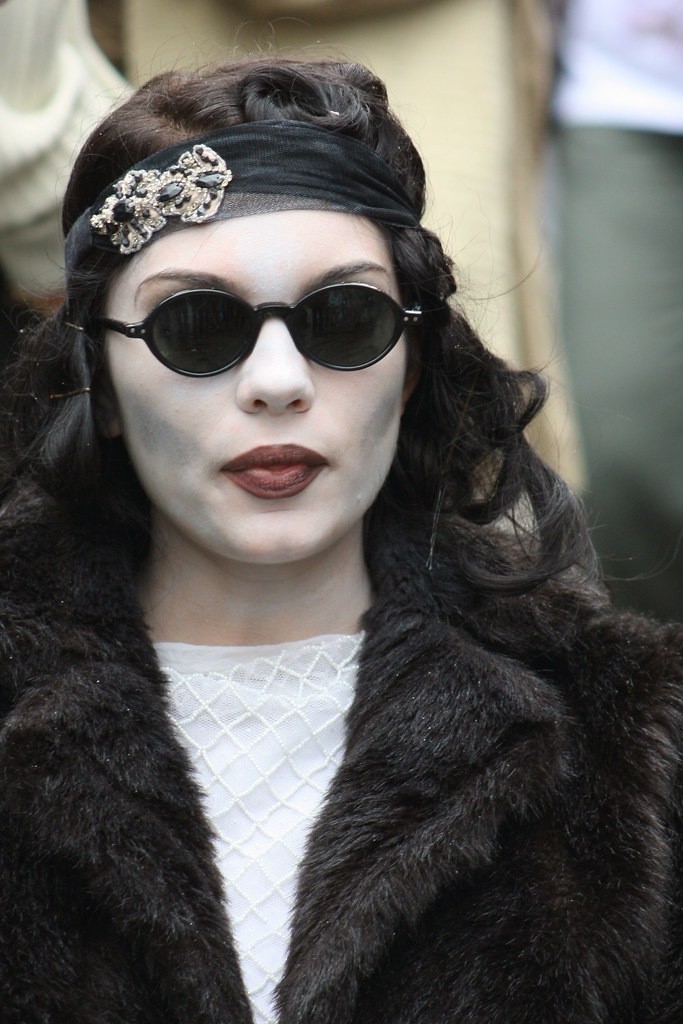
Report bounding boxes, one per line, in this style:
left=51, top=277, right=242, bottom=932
left=0, top=0, right=133, bottom=318
left=547, top=0, right=682, bottom=619
left=0, top=59, right=683, bottom=1024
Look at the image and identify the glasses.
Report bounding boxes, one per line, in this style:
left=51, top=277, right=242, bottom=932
left=96, top=283, right=424, bottom=377
